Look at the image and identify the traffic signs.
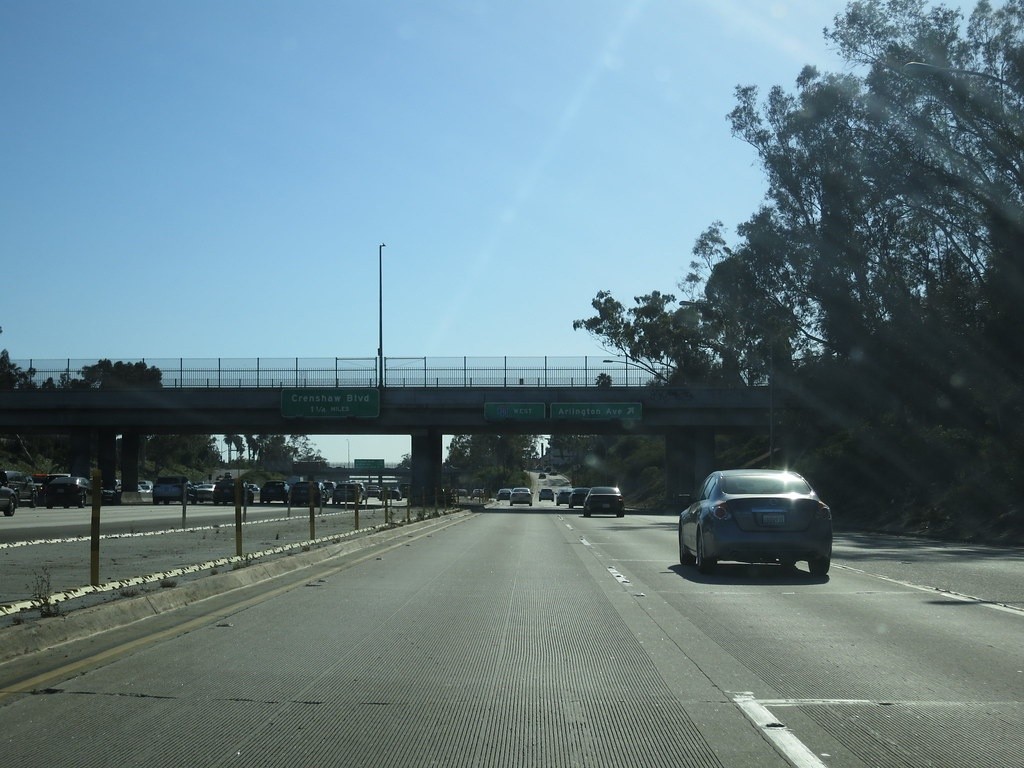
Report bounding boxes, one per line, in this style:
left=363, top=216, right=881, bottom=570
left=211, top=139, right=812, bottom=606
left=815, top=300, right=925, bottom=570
left=549, top=402, right=643, bottom=421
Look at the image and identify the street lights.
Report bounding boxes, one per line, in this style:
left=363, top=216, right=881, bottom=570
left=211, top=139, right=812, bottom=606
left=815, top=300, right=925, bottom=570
left=378, top=243, right=388, bottom=389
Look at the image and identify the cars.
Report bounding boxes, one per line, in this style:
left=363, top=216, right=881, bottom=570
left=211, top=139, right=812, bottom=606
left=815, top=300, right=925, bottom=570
left=471, top=488, right=486, bottom=498
left=555, top=489, right=573, bottom=506
left=567, top=487, right=591, bottom=508
left=676, top=470, right=834, bottom=574
left=0, top=470, right=467, bottom=518
left=539, top=467, right=558, bottom=479
left=538, top=489, right=555, bottom=502
left=496, top=489, right=512, bottom=501
left=582, top=486, right=626, bottom=517
left=508, top=488, right=534, bottom=506
